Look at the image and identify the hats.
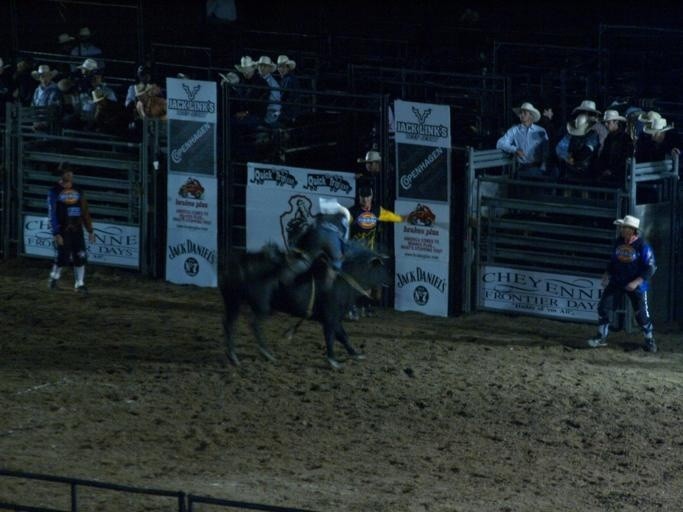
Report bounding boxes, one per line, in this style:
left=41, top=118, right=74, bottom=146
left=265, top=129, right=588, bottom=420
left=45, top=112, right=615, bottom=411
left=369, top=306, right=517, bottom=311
left=75, top=57, right=98, bottom=71
left=601, top=109, right=628, bottom=122
left=613, top=214, right=644, bottom=232
left=637, top=110, right=662, bottom=124
left=642, top=117, right=675, bottom=135
left=132, top=80, right=153, bottom=97
left=31, top=64, right=59, bottom=81
left=571, top=98, right=602, bottom=116
left=87, top=86, right=113, bottom=105
left=512, top=101, right=542, bottom=123
left=565, top=112, right=600, bottom=138
left=232, top=54, right=296, bottom=72
left=356, top=150, right=382, bottom=164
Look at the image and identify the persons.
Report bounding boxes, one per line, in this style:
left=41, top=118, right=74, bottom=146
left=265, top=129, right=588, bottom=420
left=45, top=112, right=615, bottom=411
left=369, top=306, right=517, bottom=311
left=490, top=96, right=683, bottom=224
left=354, top=149, right=391, bottom=189
left=250, top=199, right=350, bottom=319
left=1, top=26, right=167, bottom=143
left=584, top=213, right=657, bottom=353
left=343, top=182, right=407, bottom=320
left=41, top=159, right=96, bottom=297
left=218, top=55, right=306, bottom=156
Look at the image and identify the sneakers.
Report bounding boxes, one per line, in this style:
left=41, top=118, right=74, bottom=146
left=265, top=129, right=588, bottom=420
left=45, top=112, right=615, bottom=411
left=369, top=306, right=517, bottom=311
left=73, top=284, right=89, bottom=297
left=346, top=304, right=362, bottom=322
left=639, top=336, right=658, bottom=354
left=361, top=303, right=378, bottom=318
left=583, top=334, right=610, bottom=348
left=47, top=277, right=64, bottom=292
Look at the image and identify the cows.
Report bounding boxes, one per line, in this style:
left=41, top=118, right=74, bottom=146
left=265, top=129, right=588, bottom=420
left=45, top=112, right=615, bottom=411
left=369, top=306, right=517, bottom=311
left=218, top=237, right=392, bottom=372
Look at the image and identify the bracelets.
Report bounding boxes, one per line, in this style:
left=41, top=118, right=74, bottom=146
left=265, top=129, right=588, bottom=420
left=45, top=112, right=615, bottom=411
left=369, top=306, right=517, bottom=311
left=86, top=229, right=93, bottom=235
